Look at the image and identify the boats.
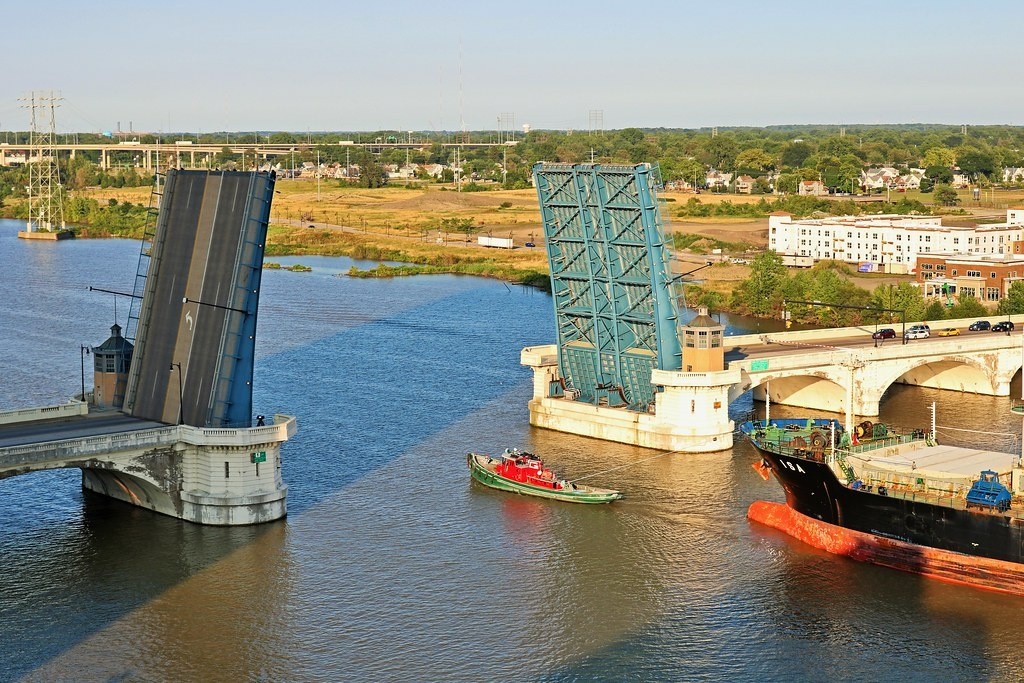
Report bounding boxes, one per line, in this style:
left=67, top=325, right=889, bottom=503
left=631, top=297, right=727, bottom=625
left=742, top=362, right=1024, bottom=596
left=463, top=449, right=622, bottom=505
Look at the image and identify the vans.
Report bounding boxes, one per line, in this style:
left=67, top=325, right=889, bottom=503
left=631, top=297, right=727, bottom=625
left=907, top=325, right=934, bottom=333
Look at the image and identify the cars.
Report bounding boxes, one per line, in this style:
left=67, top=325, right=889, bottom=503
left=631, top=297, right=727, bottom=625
left=732, top=258, right=747, bottom=264
left=994, top=321, right=1014, bottom=333
left=906, top=330, right=936, bottom=336
left=871, top=328, right=897, bottom=339
left=526, top=243, right=535, bottom=246
left=969, top=321, right=991, bottom=332
left=938, top=328, right=964, bottom=334
